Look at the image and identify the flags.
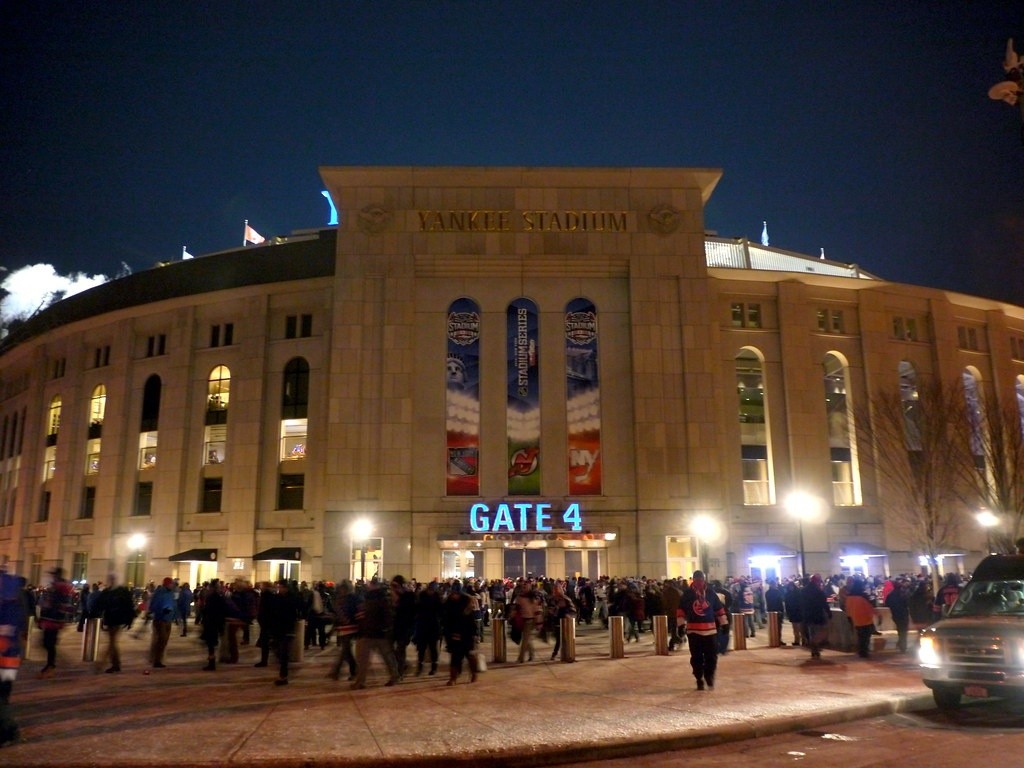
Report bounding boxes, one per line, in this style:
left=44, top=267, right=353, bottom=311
left=245, top=224, right=265, bottom=245
left=760, top=227, right=769, bottom=247
left=183, top=251, right=195, bottom=260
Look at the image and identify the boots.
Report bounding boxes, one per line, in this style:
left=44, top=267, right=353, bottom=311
left=857, top=637, right=871, bottom=658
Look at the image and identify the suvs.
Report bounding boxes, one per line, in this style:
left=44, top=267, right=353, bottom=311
left=914, top=553, right=1024, bottom=709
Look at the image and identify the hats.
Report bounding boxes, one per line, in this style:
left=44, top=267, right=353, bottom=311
left=893, top=579, right=906, bottom=589
left=811, top=574, right=822, bottom=585
left=46, top=566, right=67, bottom=582
left=694, top=571, right=703, bottom=580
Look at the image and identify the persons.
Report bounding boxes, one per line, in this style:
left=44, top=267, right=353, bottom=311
left=0, top=566, right=972, bottom=742
left=679, top=570, right=729, bottom=690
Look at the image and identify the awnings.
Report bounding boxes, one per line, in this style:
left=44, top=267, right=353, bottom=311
left=747, top=543, right=799, bottom=559
left=169, top=549, right=218, bottom=564
left=839, top=542, right=889, bottom=558
left=253, top=547, right=301, bottom=562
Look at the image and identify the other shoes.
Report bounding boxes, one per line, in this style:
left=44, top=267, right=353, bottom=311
left=104, top=664, right=121, bottom=672
left=779, top=639, right=786, bottom=646
left=625, top=634, right=641, bottom=643
left=179, top=631, right=188, bottom=637
left=325, top=659, right=481, bottom=687
left=254, top=658, right=270, bottom=668
left=745, top=634, right=755, bottom=638
left=152, top=659, right=165, bottom=669
left=792, top=641, right=807, bottom=647
left=759, top=625, right=765, bottom=629
left=202, top=658, right=218, bottom=673
left=809, top=647, right=821, bottom=658
left=697, top=678, right=715, bottom=690
left=668, top=643, right=685, bottom=651
left=273, top=676, right=289, bottom=687
left=516, top=655, right=535, bottom=662
left=549, top=653, right=558, bottom=662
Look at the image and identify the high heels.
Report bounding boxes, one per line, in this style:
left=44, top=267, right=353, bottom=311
left=41, top=664, right=56, bottom=673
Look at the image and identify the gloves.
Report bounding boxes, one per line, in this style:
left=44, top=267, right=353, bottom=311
left=679, top=624, right=686, bottom=638
left=720, top=624, right=730, bottom=638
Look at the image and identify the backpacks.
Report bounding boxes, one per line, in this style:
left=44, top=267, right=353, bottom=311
left=345, top=594, right=366, bottom=621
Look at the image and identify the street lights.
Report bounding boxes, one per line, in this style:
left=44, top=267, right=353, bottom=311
left=789, top=492, right=809, bottom=579
left=696, top=522, right=713, bottom=586
left=355, top=523, right=371, bottom=581
left=131, top=540, right=142, bottom=588
